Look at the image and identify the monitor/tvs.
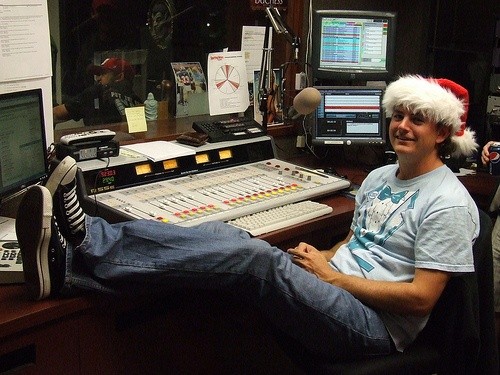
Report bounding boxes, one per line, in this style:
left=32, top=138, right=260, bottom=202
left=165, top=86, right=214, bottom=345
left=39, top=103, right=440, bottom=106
left=310, top=86, right=385, bottom=144
left=0, top=88, right=50, bottom=205
left=312, top=11, right=394, bottom=84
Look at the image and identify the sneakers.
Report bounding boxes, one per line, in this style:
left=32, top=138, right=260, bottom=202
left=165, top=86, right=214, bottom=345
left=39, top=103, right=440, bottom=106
left=47, top=157, right=85, bottom=244
left=15, top=185, right=66, bottom=298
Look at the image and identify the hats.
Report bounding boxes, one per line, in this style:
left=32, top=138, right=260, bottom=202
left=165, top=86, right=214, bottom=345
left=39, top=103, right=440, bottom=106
left=382, top=76, right=475, bottom=153
left=91, top=59, right=135, bottom=80
left=92, top=0, right=113, bottom=10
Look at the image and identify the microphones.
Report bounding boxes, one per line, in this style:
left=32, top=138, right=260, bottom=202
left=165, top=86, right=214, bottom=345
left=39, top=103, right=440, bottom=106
left=287, top=86, right=321, bottom=120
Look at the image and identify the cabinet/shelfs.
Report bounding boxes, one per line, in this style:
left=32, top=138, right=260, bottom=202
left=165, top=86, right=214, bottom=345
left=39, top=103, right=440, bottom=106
left=0, top=159, right=500, bottom=375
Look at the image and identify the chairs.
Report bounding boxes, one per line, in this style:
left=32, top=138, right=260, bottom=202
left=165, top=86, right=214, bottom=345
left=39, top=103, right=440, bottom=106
left=275, top=208, right=493, bottom=375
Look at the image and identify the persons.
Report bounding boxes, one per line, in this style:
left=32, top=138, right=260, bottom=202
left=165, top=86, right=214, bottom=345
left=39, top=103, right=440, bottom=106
left=480, top=139, right=500, bottom=313
left=14, top=74, right=481, bottom=362
left=151, top=3, right=170, bottom=40
left=173, top=66, right=205, bottom=104
left=52, top=55, right=142, bottom=128
left=266, top=71, right=279, bottom=124
left=75, top=0, right=137, bottom=126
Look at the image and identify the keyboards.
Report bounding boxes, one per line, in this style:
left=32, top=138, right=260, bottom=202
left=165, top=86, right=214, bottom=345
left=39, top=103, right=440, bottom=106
left=227, top=201, right=333, bottom=237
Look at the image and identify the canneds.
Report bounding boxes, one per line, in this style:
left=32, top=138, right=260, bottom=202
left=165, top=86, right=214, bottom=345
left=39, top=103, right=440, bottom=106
left=488, top=142, right=500, bottom=177
